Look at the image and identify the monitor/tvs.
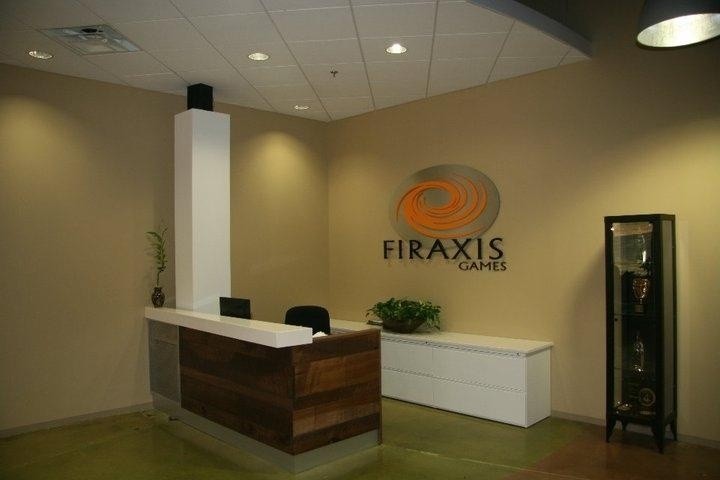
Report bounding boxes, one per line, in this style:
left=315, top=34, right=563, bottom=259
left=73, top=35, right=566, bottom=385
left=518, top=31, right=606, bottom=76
left=219, top=296, right=252, bottom=318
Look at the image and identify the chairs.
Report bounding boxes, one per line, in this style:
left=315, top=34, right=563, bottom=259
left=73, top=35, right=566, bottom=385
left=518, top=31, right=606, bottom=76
left=285, top=306, right=333, bottom=335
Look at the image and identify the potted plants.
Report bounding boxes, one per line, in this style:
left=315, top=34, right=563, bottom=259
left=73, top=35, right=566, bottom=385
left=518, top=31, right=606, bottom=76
left=145, top=218, right=171, bottom=308
left=365, top=295, right=441, bottom=333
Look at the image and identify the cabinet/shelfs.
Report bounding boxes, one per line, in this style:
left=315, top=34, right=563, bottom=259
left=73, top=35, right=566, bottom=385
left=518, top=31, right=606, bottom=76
left=606, top=215, right=677, bottom=453
left=329, top=317, right=554, bottom=428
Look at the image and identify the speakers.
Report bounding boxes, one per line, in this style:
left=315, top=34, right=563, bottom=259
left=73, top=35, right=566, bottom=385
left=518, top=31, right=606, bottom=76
left=187, top=84, right=215, bottom=111
